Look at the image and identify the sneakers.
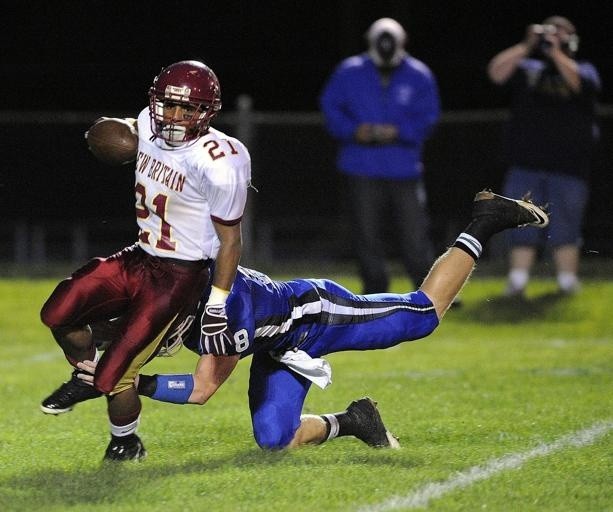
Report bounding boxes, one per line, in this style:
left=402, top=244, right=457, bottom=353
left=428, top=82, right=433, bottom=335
left=348, top=397, right=401, bottom=450
left=473, top=188, right=549, bottom=229
left=101, top=434, right=147, bottom=463
left=40, top=370, right=103, bottom=413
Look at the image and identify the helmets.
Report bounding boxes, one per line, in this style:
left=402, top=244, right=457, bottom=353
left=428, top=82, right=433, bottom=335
left=108, top=307, right=199, bottom=358
left=148, top=61, right=223, bottom=141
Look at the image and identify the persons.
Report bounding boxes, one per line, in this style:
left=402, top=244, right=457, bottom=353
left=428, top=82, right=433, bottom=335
left=40, top=57, right=253, bottom=466
left=484, top=13, right=602, bottom=302
left=319, top=13, right=461, bottom=312
left=75, top=188, right=550, bottom=454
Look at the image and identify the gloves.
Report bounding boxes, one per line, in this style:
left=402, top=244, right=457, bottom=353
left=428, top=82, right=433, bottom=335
left=77, top=359, right=155, bottom=399
left=200, top=286, right=235, bottom=358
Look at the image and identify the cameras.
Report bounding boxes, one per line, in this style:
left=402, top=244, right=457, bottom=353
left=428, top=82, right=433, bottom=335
left=536, top=33, right=569, bottom=49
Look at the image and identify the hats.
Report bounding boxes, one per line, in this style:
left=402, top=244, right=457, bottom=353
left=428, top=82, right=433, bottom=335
left=370, top=18, right=407, bottom=66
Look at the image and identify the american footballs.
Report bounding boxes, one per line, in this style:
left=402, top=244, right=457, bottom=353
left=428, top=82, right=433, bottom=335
left=88, top=120, right=138, bottom=166
